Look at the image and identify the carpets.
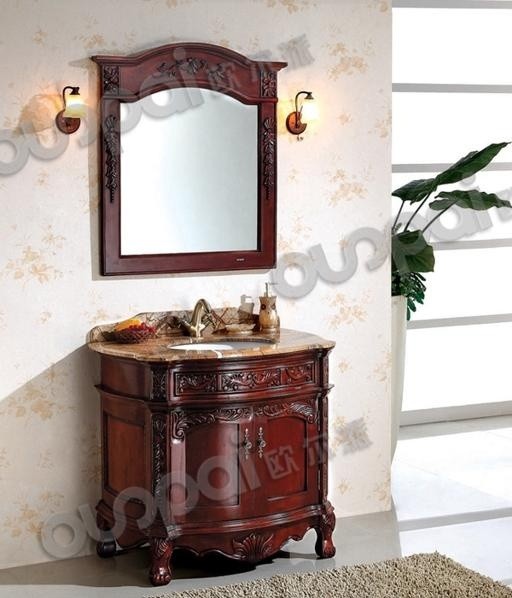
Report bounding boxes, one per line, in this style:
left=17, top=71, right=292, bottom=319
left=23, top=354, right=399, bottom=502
left=140, top=549, right=512, bottom=598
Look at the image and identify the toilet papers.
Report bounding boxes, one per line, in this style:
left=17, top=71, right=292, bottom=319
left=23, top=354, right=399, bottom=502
left=113, top=318, right=141, bottom=332
left=238, top=294, right=254, bottom=323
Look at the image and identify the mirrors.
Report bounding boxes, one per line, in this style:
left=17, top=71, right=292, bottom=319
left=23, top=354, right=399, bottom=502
left=89, top=42, right=288, bottom=276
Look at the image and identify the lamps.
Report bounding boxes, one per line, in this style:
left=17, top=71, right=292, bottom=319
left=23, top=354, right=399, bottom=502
left=56, top=86, right=87, bottom=135
left=286, top=91, right=317, bottom=141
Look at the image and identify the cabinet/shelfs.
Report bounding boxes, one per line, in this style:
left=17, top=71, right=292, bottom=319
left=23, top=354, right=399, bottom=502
left=86, top=307, right=337, bottom=586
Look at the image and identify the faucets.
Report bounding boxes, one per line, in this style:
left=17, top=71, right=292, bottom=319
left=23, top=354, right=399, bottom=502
left=176, top=299, right=212, bottom=337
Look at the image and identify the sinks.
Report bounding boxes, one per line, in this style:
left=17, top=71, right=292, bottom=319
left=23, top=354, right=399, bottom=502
left=166, top=336, right=276, bottom=351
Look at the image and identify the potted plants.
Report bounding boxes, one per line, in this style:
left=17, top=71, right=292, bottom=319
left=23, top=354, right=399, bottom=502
left=390, top=142, right=512, bottom=463
